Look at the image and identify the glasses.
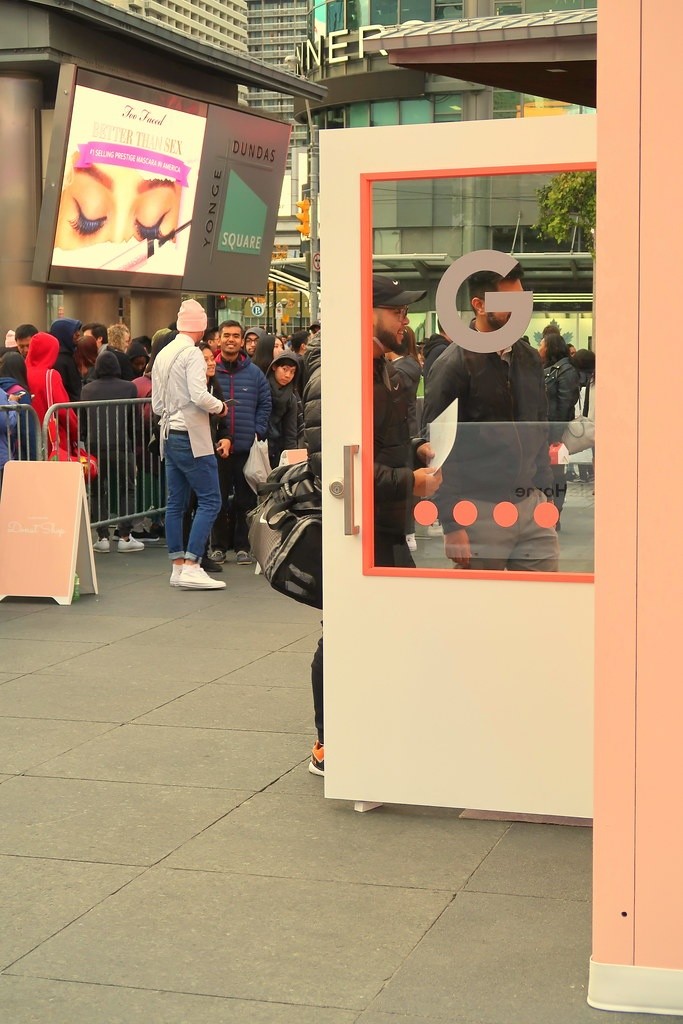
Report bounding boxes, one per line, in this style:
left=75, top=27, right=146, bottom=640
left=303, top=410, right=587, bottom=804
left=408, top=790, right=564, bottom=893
left=209, top=336, right=220, bottom=343
left=373, top=303, right=409, bottom=322
left=245, top=337, right=260, bottom=345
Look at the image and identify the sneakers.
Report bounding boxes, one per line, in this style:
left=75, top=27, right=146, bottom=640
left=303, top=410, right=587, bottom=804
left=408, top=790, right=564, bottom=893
left=200, top=552, right=223, bottom=571
left=112, top=529, right=120, bottom=539
left=211, top=550, right=226, bottom=564
left=308, top=740, right=325, bottom=776
left=236, top=550, right=252, bottom=565
left=117, top=534, right=144, bottom=552
left=169, top=570, right=184, bottom=586
left=131, top=528, right=160, bottom=542
left=92, top=536, right=111, bottom=553
left=178, top=567, right=226, bottom=589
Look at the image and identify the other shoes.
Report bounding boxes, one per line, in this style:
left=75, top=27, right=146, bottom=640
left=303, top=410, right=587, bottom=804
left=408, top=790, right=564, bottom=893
left=564, top=470, right=595, bottom=484
left=149, top=523, right=166, bottom=539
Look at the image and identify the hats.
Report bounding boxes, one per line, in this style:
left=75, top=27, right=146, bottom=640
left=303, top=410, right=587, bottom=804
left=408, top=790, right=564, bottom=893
left=175, top=299, right=207, bottom=332
left=372, top=274, right=427, bottom=307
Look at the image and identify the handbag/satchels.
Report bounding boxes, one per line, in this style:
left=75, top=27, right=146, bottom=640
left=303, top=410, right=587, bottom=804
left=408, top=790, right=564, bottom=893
left=48, top=443, right=98, bottom=484
left=245, top=458, right=323, bottom=613
left=560, top=415, right=596, bottom=455
left=242, top=433, right=273, bottom=494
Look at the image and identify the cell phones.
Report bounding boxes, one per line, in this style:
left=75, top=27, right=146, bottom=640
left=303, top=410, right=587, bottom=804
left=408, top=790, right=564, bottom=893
left=215, top=443, right=223, bottom=455
left=225, top=400, right=239, bottom=407
left=16, top=392, right=26, bottom=397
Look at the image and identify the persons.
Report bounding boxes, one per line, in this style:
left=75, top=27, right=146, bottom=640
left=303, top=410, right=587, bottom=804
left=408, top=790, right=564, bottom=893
left=56, top=149, right=181, bottom=249
left=420, top=261, right=560, bottom=572
left=0, top=254, right=594, bottom=592
left=302, top=274, right=443, bottom=775
